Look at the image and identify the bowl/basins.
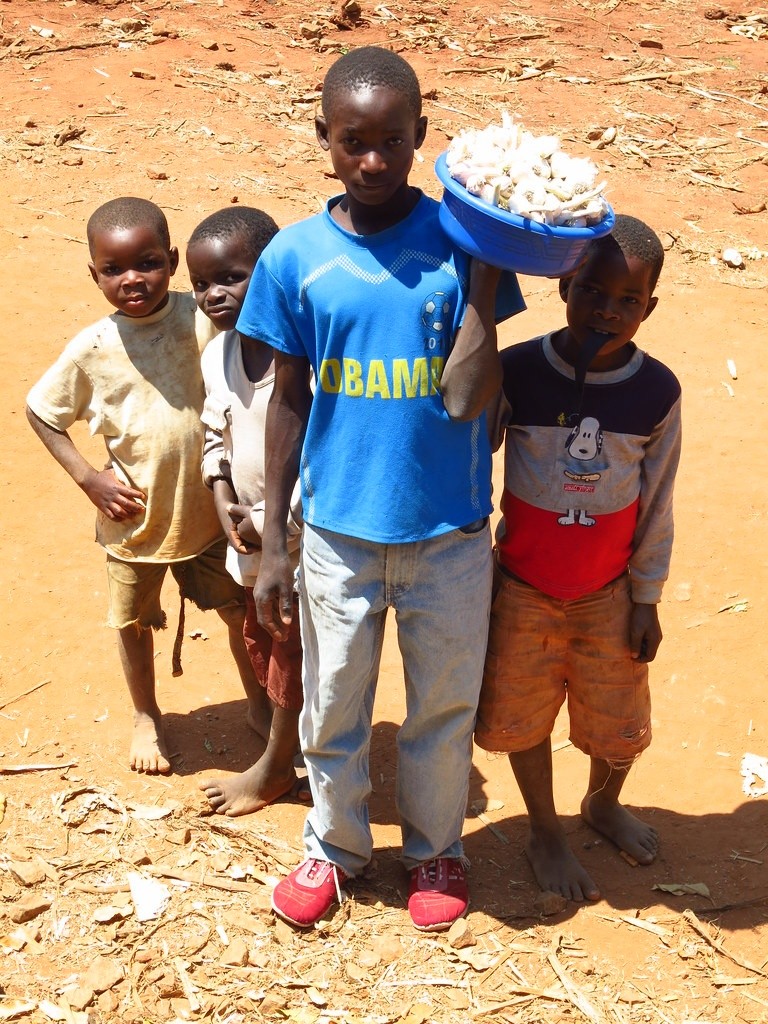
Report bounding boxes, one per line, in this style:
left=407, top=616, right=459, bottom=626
left=434, top=161, right=617, bottom=274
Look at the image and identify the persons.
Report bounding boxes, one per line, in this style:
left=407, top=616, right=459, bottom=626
left=473, top=215, right=682, bottom=901
left=26, top=197, right=271, bottom=772
left=187, top=206, right=353, bottom=816
left=236, top=48, right=527, bottom=930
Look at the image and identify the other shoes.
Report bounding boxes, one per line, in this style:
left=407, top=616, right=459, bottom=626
left=272, top=856, right=344, bottom=927
left=407, top=858, right=471, bottom=929
